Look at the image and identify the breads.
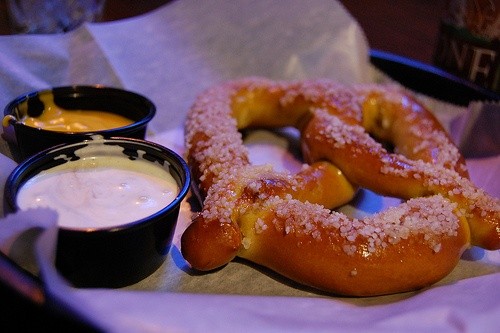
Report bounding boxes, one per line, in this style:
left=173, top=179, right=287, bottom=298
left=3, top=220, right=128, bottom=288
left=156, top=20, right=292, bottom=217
left=180, top=77, right=500, bottom=297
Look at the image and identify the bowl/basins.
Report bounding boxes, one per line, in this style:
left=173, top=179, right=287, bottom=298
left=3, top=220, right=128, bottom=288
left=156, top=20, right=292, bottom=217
left=5, top=135, right=191, bottom=289
left=4, top=84, right=157, bottom=165
